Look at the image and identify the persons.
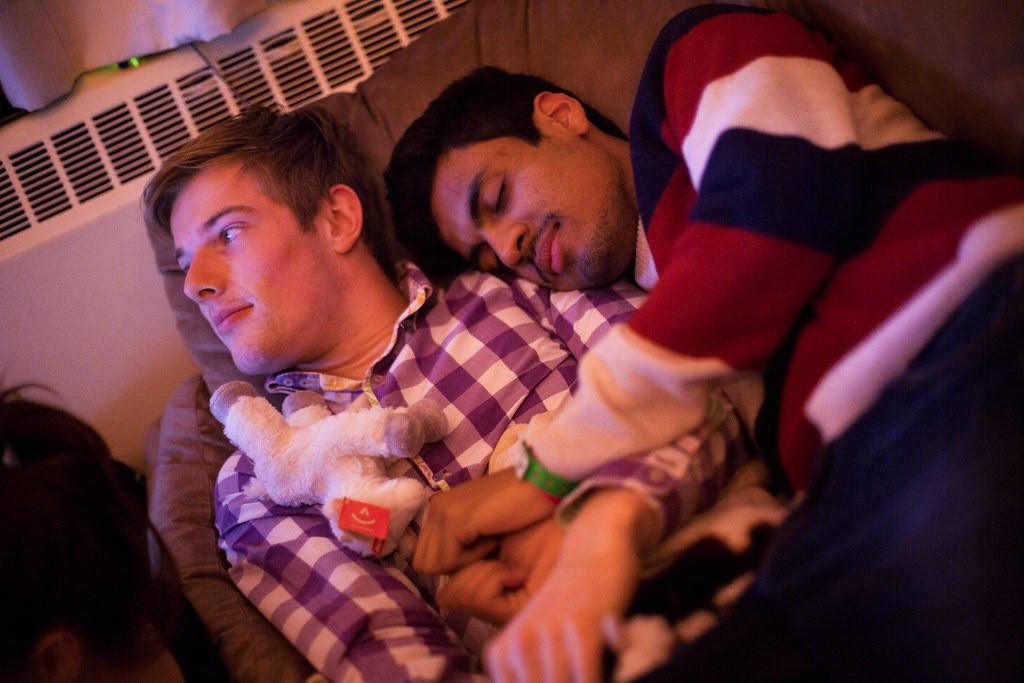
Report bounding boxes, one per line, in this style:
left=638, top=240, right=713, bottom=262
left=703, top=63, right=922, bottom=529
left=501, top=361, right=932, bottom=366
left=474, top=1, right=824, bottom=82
left=141, top=0, right=1024, bottom=683
left=0, top=381, right=128, bottom=682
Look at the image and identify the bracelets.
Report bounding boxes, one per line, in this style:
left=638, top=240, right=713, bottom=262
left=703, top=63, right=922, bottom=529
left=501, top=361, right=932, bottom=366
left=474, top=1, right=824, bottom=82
left=510, top=441, right=584, bottom=515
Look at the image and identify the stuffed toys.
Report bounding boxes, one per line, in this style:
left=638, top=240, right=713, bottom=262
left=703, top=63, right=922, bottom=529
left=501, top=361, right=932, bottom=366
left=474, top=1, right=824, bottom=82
left=209, top=379, right=449, bottom=560
